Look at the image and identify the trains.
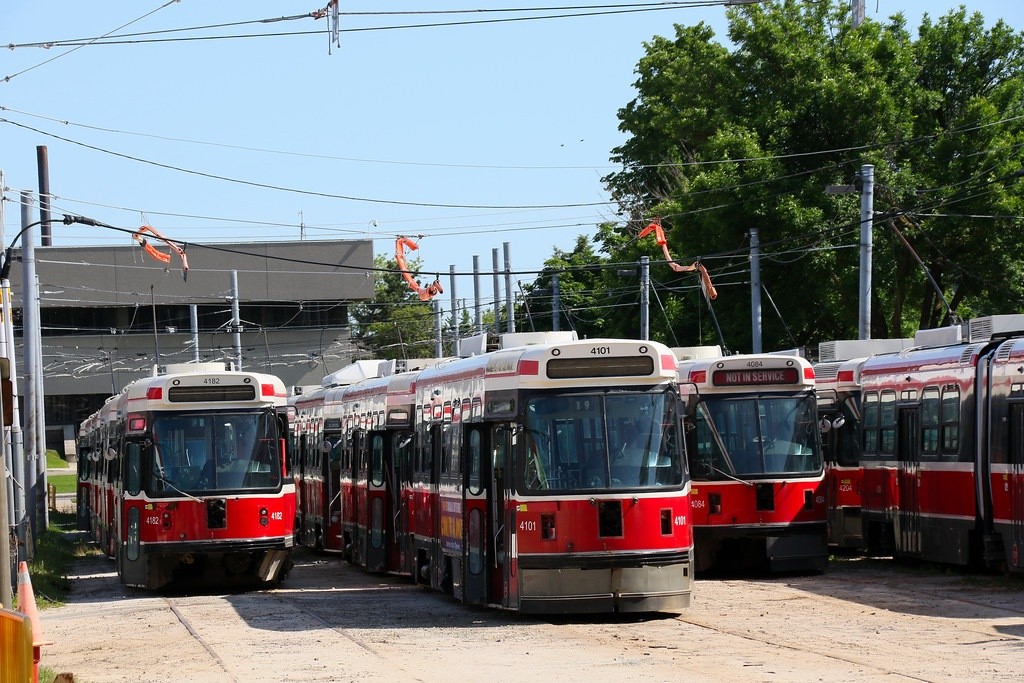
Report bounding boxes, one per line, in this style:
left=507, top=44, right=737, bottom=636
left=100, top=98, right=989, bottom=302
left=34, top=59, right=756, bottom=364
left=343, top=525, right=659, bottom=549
left=798, top=306, right=1024, bottom=586
left=73, top=358, right=307, bottom=592
left=655, top=339, right=844, bottom=579
left=268, top=319, right=704, bottom=623
